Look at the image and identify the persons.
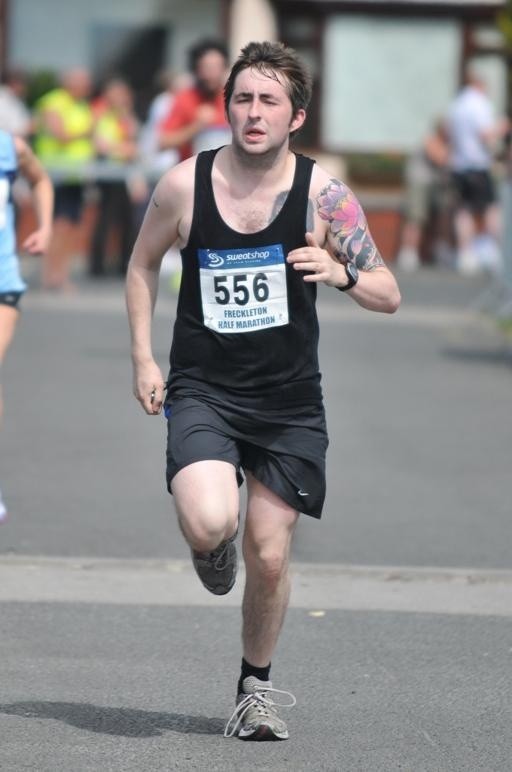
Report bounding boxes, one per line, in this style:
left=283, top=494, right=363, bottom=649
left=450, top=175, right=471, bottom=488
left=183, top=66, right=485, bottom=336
left=401, top=52, right=508, bottom=279
left=1, top=61, right=142, bottom=279
left=0, top=128, right=59, bottom=523
left=136, top=37, right=232, bottom=179
left=123, top=31, right=408, bottom=739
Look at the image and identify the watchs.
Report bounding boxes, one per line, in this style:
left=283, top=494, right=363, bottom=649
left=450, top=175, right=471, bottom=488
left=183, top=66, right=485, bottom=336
left=331, top=263, right=358, bottom=294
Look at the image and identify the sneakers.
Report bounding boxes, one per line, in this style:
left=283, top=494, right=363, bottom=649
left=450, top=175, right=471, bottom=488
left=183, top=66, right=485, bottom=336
left=188, top=512, right=242, bottom=595
left=236, top=674, right=288, bottom=741
